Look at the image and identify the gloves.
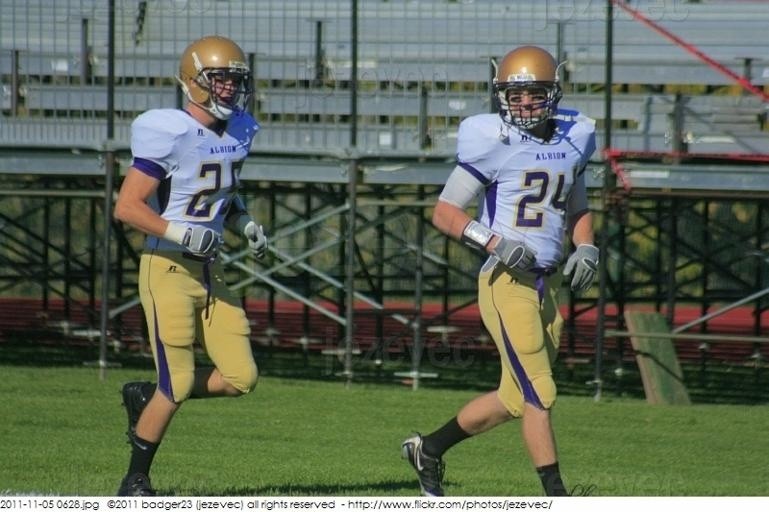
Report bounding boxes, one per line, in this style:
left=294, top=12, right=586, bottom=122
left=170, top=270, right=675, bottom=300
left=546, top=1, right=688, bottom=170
left=561, top=243, right=601, bottom=294
left=228, top=212, right=274, bottom=260
left=491, top=232, right=539, bottom=274
left=164, top=216, right=226, bottom=264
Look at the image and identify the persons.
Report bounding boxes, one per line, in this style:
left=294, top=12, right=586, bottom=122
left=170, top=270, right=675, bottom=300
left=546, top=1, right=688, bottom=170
left=396, top=46, right=608, bottom=496
left=108, top=30, right=270, bottom=496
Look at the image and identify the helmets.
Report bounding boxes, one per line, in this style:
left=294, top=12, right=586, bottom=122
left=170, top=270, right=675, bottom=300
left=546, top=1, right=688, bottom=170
left=172, top=34, right=251, bottom=120
left=489, top=45, right=567, bottom=132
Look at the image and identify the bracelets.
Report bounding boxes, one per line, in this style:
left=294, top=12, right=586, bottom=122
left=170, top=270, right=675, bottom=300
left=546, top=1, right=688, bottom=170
left=458, top=220, right=495, bottom=253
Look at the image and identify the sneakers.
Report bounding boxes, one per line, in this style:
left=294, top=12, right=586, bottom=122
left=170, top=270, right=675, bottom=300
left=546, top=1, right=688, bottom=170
left=113, top=471, right=156, bottom=496
left=396, top=432, right=446, bottom=497
left=121, top=379, right=149, bottom=447
